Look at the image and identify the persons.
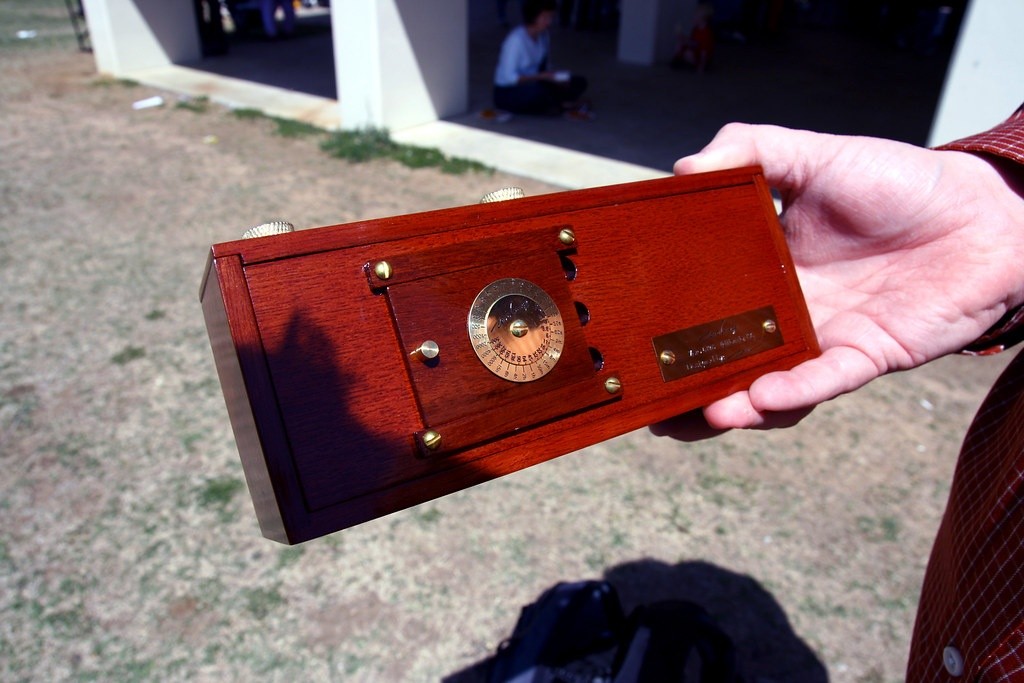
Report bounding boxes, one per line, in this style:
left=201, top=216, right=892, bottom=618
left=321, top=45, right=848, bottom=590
left=491, top=1, right=585, bottom=119
left=648, top=93, right=1024, bottom=683
left=670, top=5, right=714, bottom=75
